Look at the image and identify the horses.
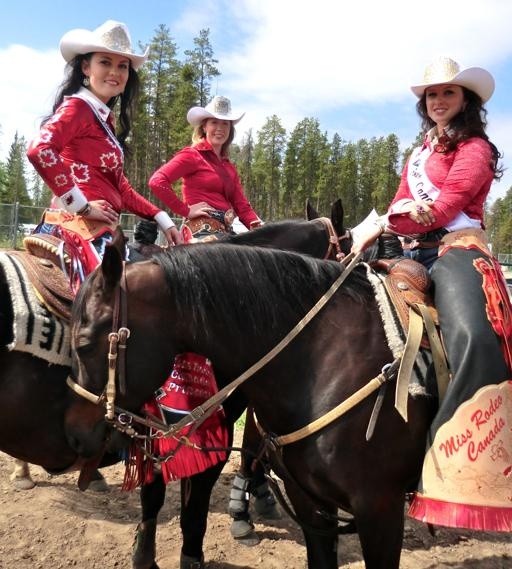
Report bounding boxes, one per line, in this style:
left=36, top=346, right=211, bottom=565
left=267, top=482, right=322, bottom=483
left=64, top=224, right=512, bottom=569
left=0, top=197, right=354, bottom=549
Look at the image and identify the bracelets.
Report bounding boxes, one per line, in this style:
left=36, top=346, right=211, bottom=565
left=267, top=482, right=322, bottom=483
left=76, top=204, right=92, bottom=216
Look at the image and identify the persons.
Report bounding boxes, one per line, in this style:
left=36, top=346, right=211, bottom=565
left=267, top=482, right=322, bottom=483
left=375, top=55, right=512, bottom=532
left=127, top=350, right=230, bottom=568
left=147, top=96, right=262, bottom=245
left=25, top=20, right=182, bottom=323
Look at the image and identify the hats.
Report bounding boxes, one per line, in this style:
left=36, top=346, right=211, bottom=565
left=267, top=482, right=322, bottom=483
left=59, top=20, right=150, bottom=70
left=186, top=96, right=246, bottom=129
left=410, top=58, right=495, bottom=105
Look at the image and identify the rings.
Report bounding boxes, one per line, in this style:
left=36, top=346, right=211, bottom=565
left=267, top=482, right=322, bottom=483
left=415, top=204, right=426, bottom=215
left=104, top=204, right=109, bottom=211
